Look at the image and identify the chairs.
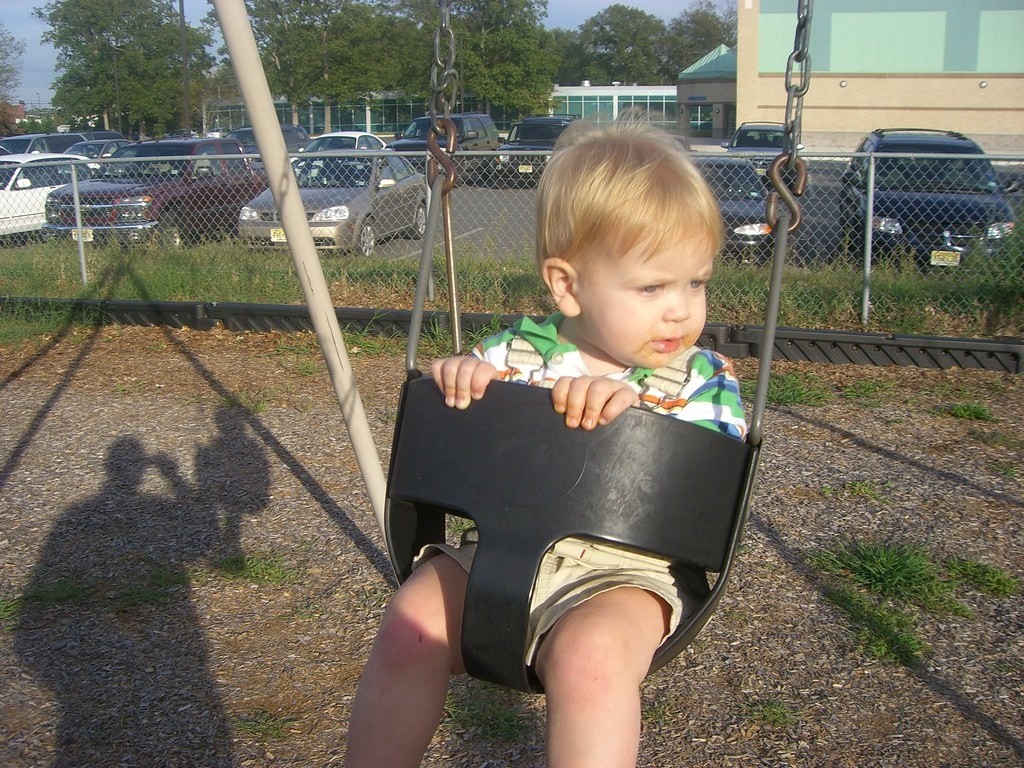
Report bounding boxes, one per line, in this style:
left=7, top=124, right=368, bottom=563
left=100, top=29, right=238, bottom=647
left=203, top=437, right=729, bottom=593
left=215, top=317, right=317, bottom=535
left=772, top=135, right=783, bottom=145
left=744, top=136, right=755, bottom=144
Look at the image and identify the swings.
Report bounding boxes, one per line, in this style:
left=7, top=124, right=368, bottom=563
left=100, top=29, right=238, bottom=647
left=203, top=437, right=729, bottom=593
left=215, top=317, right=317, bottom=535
left=376, top=0, right=819, bottom=698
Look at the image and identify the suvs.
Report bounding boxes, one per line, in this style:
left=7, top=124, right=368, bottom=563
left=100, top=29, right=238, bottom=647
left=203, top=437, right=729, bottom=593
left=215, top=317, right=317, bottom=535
left=68, top=128, right=126, bottom=141
left=221, top=123, right=313, bottom=154
left=493, top=113, right=580, bottom=189
left=0, top=131, right=90, bottom=155
left=721, top=121, right=803, bottom=181
left=386, top=111, right=500, bottom=188
left=839, top=127, right=1022, bottom=269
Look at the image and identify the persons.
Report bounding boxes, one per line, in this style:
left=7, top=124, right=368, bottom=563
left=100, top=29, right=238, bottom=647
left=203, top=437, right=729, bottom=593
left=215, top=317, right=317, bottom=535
left=344, top=121, right=747, bottom=768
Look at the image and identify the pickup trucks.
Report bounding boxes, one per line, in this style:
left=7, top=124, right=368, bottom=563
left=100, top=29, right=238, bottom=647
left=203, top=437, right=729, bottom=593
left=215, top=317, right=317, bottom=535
left=42, top=137, right=267, bottom=252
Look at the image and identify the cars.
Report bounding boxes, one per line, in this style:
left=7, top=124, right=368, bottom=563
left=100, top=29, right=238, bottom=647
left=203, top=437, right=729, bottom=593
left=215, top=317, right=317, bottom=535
left=237, top=149, right=427, bottom=257
left=290, top=130, right=395, bottom=180
left=0, top=152, right=121, bottom=235
left=57, top=139, right=137, bottom=177
left=687, top=156, right=776, bottom=263
